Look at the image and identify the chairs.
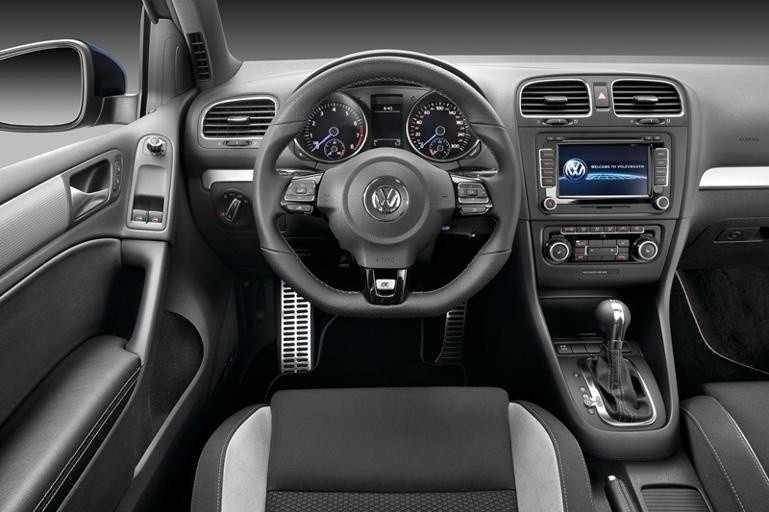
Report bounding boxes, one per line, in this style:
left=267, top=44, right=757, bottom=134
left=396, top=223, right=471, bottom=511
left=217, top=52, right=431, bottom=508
left=680, top=381, right=769, bottom=512
left=190, top=386, right=596, bottom=512
